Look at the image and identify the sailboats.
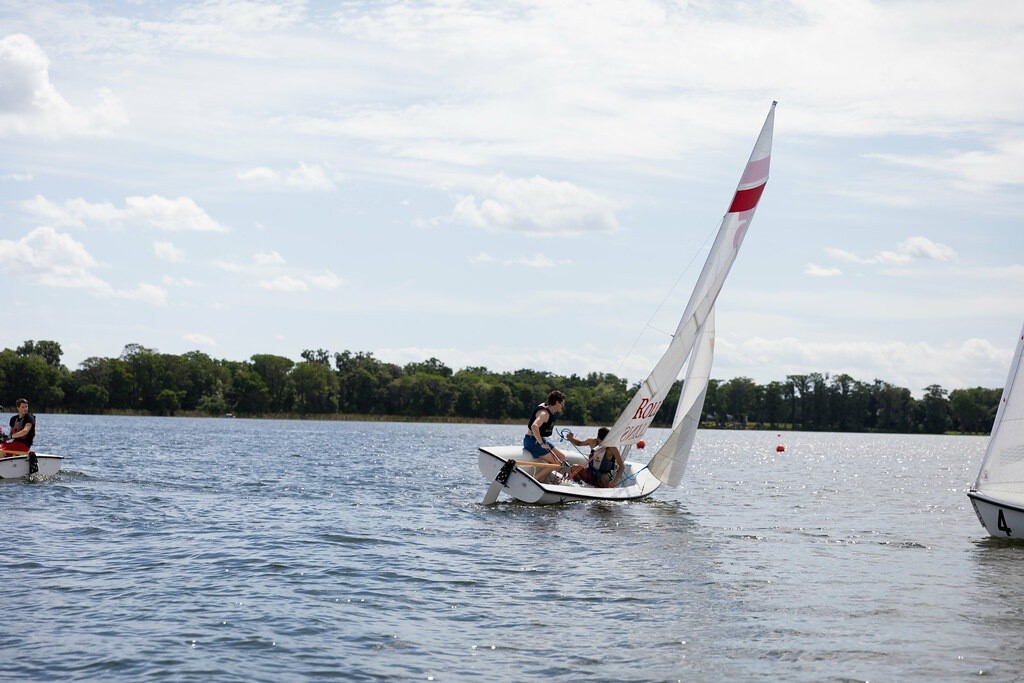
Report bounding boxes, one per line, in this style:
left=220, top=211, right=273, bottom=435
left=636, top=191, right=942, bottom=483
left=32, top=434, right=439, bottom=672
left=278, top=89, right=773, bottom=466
left=477, top=100, right=779, bottom=508
left=966, top=324, right=1023, bottom=548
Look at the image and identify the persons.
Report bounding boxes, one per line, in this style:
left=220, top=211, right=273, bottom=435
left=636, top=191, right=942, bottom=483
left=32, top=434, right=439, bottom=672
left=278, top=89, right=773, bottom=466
left=0, top=399, right=36, bottom=458
left=567, top=428, right=625, bottom=488
left=523, top=391, right=566, bottom=484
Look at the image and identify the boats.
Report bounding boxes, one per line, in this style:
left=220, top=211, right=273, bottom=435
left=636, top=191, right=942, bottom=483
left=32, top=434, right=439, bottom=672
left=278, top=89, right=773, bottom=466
left=0, top=449, right=65, bottom=481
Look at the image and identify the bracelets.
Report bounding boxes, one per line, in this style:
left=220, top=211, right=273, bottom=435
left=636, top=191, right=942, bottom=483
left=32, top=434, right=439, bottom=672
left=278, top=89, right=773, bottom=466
left=11, top=435, right=12, bottom=439
left=540, top=443, right=544, bottom=445
